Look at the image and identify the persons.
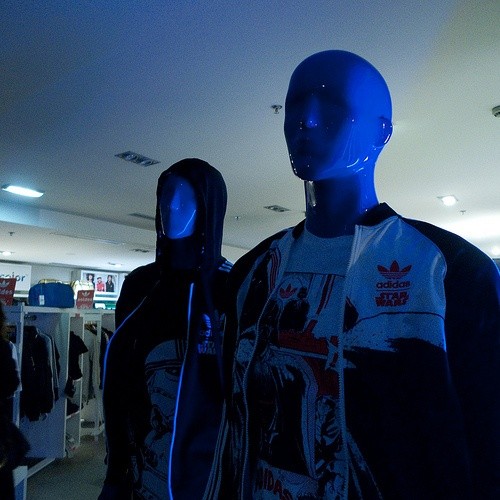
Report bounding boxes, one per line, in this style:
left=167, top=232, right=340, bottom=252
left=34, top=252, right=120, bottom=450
left=204, top=51, right=500, bottom=500
left=86, top=273, right=115, bottom=292
left=98, top=158, right=235, bottom=500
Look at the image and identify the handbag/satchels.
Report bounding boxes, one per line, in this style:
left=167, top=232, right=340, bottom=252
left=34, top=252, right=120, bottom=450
left=28, top=280, right=74, bottom=308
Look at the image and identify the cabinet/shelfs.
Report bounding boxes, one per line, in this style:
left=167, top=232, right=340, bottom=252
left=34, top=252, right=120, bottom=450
left=2, top=301, right=116, bottom=477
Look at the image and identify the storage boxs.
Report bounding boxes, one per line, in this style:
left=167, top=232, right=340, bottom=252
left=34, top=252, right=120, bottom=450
left=28, top=283, right=75, bottom=308
